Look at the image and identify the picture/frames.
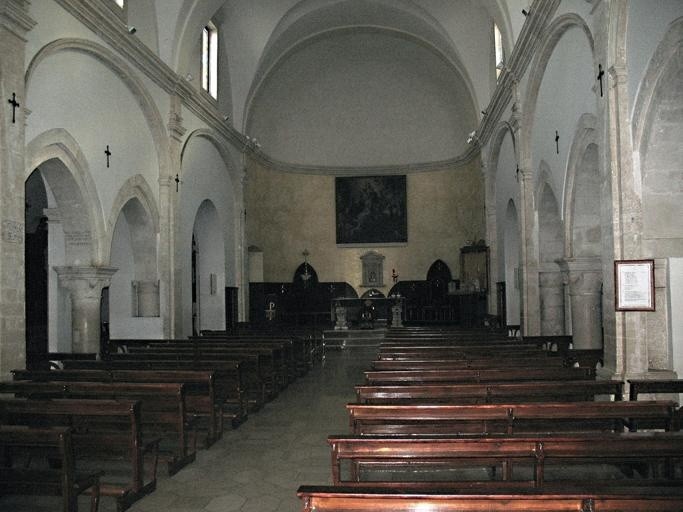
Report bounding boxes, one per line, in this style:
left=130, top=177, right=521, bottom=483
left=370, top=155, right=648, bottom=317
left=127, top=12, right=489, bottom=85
left=614, top=260, right=656, bottom=312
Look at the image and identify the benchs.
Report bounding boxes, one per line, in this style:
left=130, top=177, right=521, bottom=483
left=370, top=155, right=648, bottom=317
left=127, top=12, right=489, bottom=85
left=0, top=321, right=316, bottom=512
left=298, top=327, right=683, bottom=512
